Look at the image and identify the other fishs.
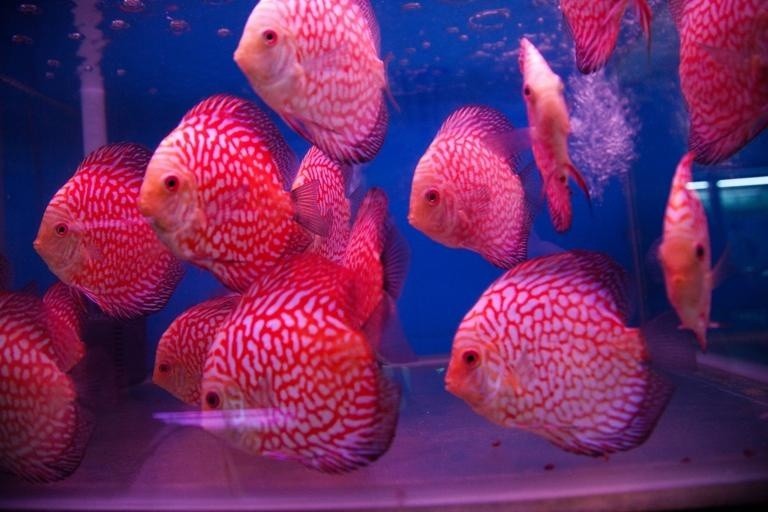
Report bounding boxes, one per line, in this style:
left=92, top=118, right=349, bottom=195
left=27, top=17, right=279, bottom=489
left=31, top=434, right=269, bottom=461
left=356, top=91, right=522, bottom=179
left=0, top=96, right=409, bottom=484
